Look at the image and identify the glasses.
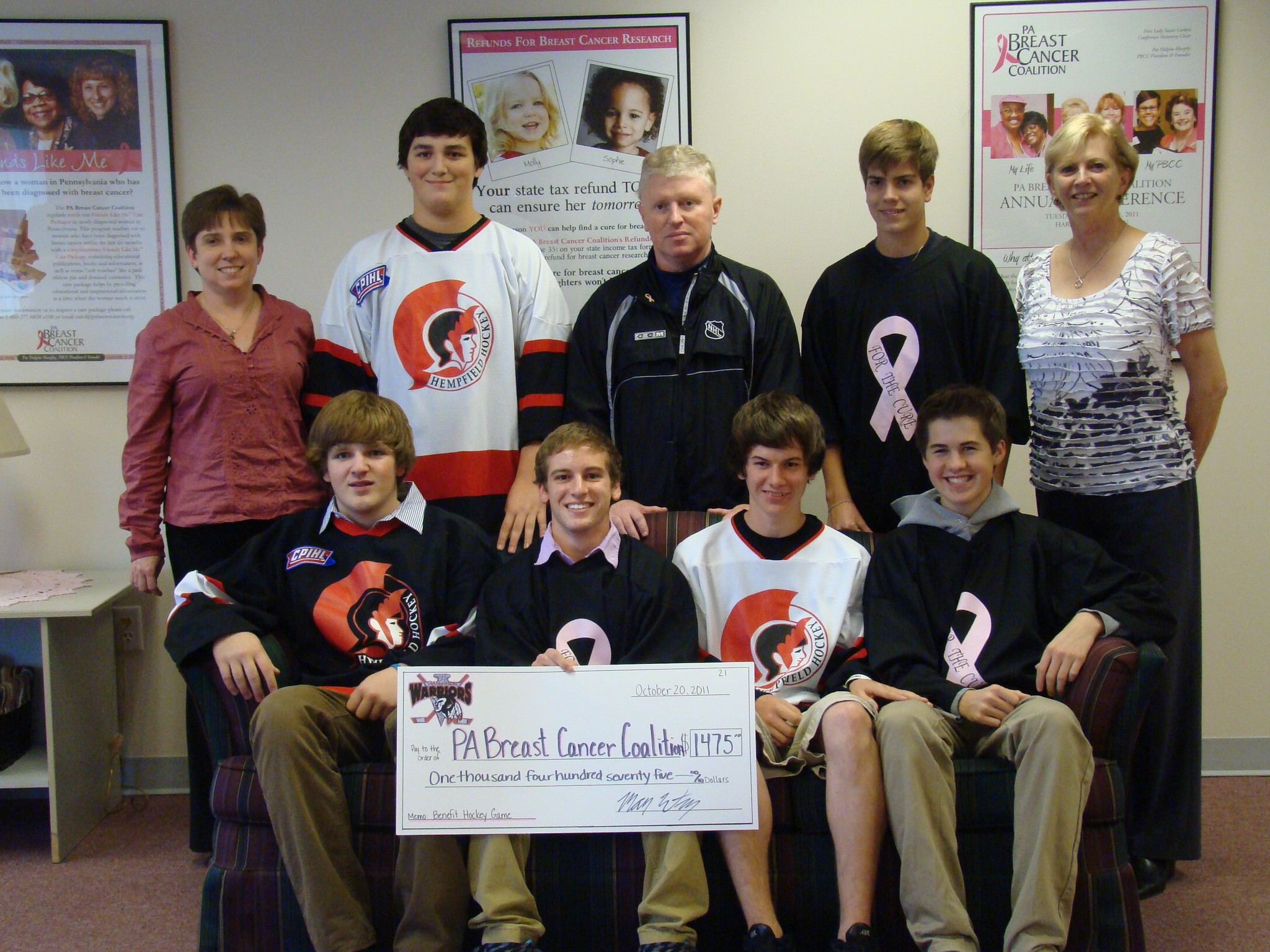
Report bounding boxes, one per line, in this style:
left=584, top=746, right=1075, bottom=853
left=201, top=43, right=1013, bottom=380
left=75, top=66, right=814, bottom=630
left=20, top=89, right=56, bottom=104
left=1138, top=106, right=1158, bottom=112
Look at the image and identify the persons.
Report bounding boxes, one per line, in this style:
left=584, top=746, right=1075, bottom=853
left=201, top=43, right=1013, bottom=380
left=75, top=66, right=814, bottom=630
left=163, top=387, right=497, bottom=951
left=310, top=95, right=572, bottom=571
left=862, top=385, right=1179, bottom=952
left=581, top=63, right=666, bottom=160
left=565, top=138, right=806, bottom=542
left=478, top=69, right=561, bottom=166
left=0, top=51, right=142, bottom=160
left=1006, top=115, right=1229, bottom=906
left=457, top=420, right=712, bottom=952
left=983, top=90, right=1201, bottom=167
left=810, top=116, right=1031, bottom=570
left=669, top=386, right=933, bottom=952
left=118, top=185, right=320, bottom=862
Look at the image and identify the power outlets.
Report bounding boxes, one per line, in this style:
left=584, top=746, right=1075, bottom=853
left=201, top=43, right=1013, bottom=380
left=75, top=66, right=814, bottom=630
left=113, top=605, right=144, bottom=652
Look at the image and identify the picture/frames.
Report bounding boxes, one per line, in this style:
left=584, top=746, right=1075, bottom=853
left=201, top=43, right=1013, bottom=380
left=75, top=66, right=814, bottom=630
left=447, top=11, right=692, bottom=329
left=969, top=0, right=1219, bottom=362
left=0, top=19, right=181, bottom=386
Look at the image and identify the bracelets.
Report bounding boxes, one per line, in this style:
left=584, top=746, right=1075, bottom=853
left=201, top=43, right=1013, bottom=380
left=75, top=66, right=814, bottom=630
left=390, top=662, right=402, bottom=673
left=827, top=500, right=854, bottom=514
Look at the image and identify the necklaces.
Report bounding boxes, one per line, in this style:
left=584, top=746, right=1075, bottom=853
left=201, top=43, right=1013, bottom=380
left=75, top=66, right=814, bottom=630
left=197, top=290, right=256, bottom=341
left=1067, top=222, right=1126, bottom=289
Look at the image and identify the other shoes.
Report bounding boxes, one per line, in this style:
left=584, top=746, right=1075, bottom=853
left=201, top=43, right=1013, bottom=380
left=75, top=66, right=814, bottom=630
left=831, top=922, right=874, bottom=952
left=748, top=923, right=787, bottom=952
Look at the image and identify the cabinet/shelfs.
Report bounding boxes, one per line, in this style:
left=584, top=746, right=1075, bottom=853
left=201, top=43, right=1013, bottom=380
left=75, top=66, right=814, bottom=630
left=0, top=570, right=135, bottom=865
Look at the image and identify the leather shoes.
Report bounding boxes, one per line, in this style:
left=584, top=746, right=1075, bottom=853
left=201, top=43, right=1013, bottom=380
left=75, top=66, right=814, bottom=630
left=1130, top=857, right=1177, bottom=899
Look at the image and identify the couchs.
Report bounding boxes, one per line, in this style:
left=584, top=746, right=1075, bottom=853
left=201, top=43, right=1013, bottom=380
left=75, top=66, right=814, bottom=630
left=198, top=511, right=1169, bottom=952
